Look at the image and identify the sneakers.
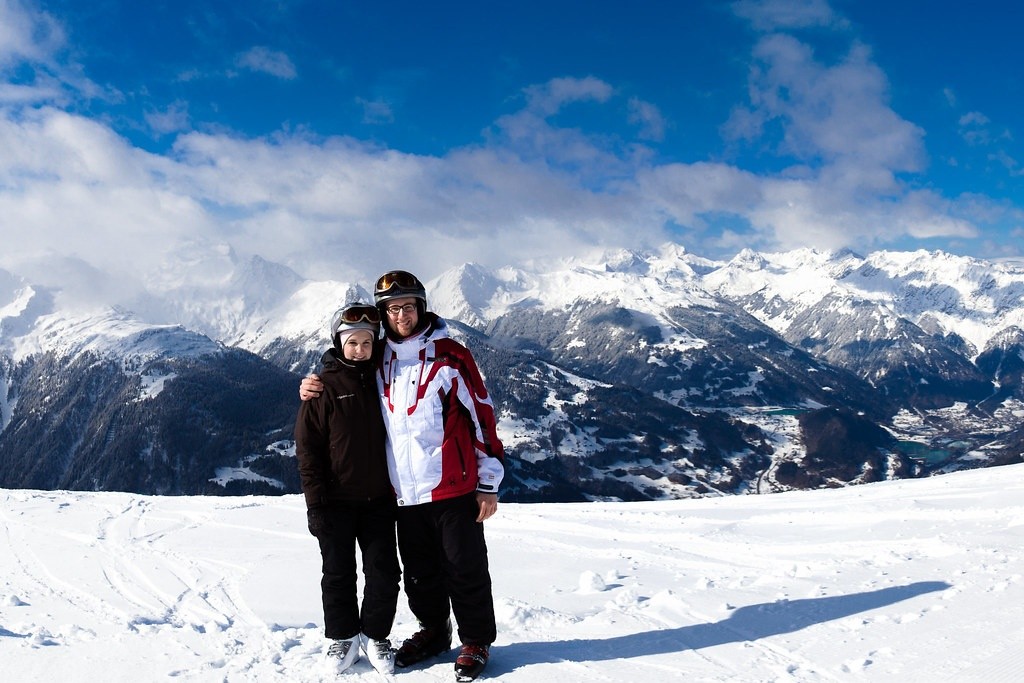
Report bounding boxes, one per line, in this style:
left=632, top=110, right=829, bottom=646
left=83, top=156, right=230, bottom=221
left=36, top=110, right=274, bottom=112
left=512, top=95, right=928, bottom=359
left=454, top=643, right=491, bottom=683
left=324, top=632, right=395, bottom=674
left=394, top=615, right=452, bottom=671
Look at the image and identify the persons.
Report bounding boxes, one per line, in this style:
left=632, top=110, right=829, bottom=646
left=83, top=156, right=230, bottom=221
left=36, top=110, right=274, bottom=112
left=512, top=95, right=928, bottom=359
left=294, top=303, right=403, bottom=675
left=300, top=270, right=506, bottom=683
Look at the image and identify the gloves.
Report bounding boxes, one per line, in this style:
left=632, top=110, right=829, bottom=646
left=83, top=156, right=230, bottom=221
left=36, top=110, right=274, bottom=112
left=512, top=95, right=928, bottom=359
left=307, top=506, right=328, bottom=536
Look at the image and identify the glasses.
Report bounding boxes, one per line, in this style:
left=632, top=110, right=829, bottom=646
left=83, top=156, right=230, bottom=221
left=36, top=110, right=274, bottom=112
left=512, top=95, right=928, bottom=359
left=341, top=305, right=380, bottom=324
left=374, top=271, right=424, bottom=295
left=386, top=302, right=418, bottom=314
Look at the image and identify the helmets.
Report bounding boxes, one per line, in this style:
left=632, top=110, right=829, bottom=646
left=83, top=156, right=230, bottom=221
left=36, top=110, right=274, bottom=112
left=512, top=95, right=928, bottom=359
left=374, top=270, right=427, bottom=308
left=331, top=302, right=380, bottom=351
